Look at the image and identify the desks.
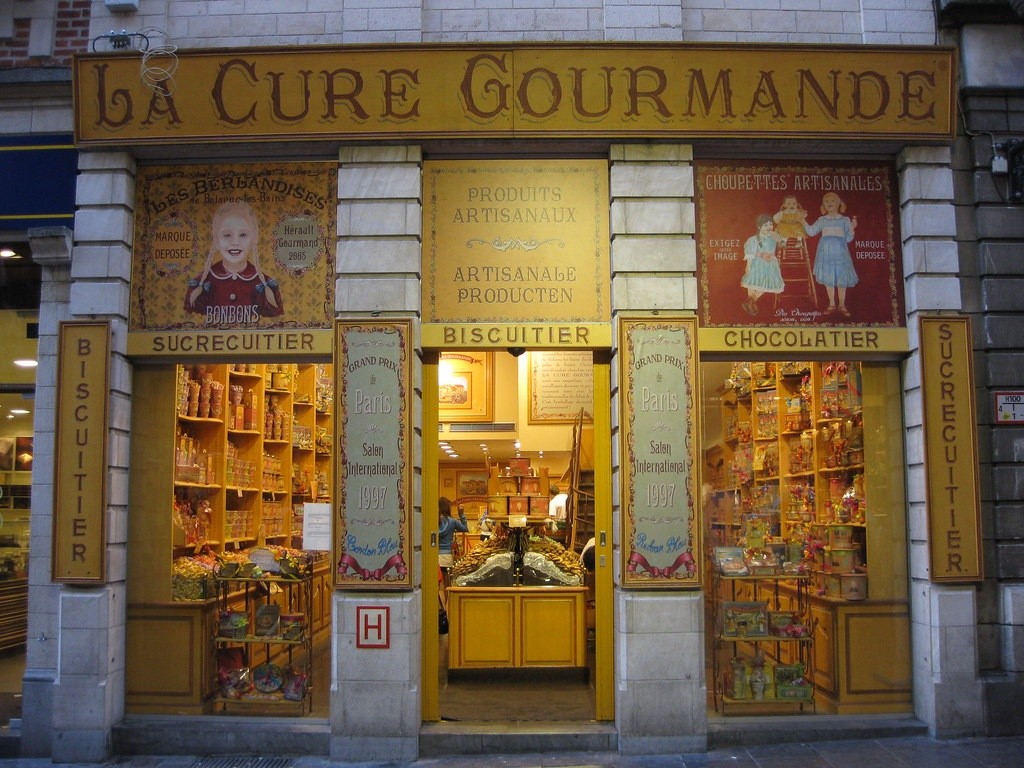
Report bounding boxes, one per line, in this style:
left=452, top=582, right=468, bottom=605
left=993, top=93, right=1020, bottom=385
left=445, top=585, right=588, bottom=669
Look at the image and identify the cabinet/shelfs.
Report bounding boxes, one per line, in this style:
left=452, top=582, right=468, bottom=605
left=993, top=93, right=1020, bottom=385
left=703, top=362, right=913, bottom=713
left=209, top=562, right=313, bottom=718
left=711, top=573, right=817, bottom=716
left=125, top=361, right=332, bottom=717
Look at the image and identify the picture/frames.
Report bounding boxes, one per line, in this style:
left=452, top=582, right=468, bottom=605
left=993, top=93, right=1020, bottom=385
left=437, top=351, right=495, bottom=423
left=526, top=352, right=593, bottom=425
left=330, top=315, right=414, bottom=590
left=617, top=315, right=706, bottom=592
left=919, top=311, right=987, bottom=587
left=48, top=320, right=113, bottom=589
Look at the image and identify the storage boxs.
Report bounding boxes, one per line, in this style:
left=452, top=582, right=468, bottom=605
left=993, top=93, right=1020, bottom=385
left=519, top=476, right=541, bottom=495
left=510, top=458, right=531, bottom=477
left=787, top=421, right=808, bottom=430
left=530, top=496, right=549, bottom=516
left=509, top=495, right=529, bottom=516
left=487, top=495, right=508, bottom=516
left=785, top=413, right=809, bottom=421
left=496, top=475, right=518, bottom=494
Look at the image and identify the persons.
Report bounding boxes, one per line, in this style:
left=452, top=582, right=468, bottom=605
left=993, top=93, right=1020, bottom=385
left=548, top=485, right=568, bottom=529
left=184, top=200, right=283, bottom=320
left=579, top=536, right=596, bottom=571
left=477, top=512, right=494, bottom=542
left=438, top=497, right=468, bottom=614
